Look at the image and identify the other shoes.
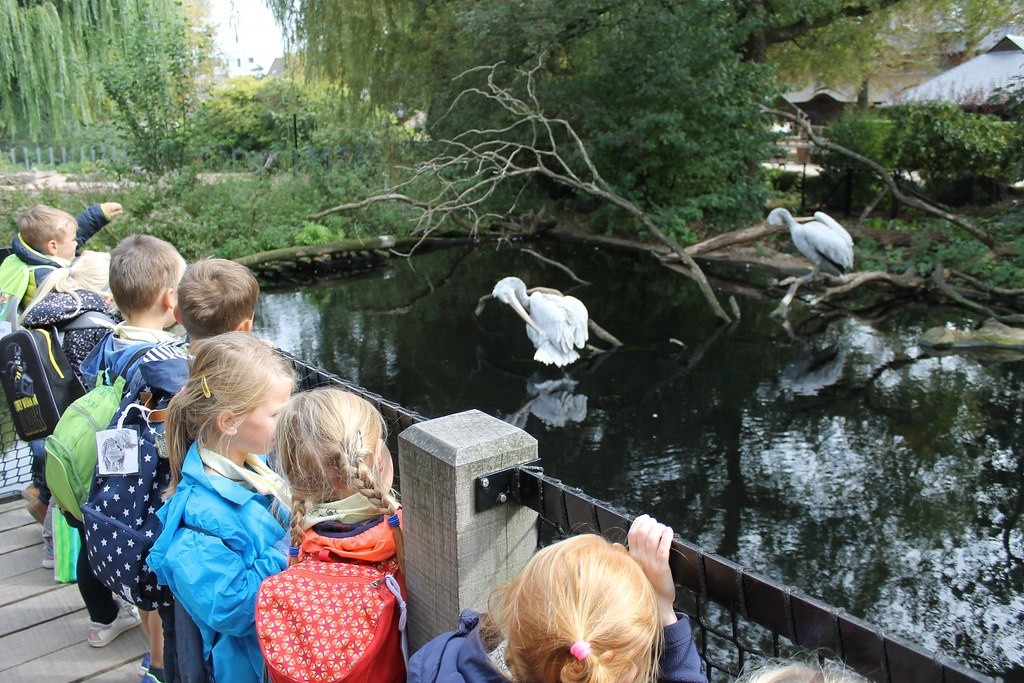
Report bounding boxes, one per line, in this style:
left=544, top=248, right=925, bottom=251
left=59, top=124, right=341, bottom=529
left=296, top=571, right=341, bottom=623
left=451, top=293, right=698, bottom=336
left=26, top=498, right=48, bottom=525
left=141, top=672, right=162, bottom=683
left=21, top=484, right=40, bottom=503
left=137, top=649, right=151, bottom=676
left=42, top=545, right=55, bottom=568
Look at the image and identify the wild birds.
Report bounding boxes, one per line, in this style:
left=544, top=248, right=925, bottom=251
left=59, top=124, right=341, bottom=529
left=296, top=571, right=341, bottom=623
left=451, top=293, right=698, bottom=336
left=768, top=208, right=854, bottom=282
left=493, top=276, right=588, bottom=367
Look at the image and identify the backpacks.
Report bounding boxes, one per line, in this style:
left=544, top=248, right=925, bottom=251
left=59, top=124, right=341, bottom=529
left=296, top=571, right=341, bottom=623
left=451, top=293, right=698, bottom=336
left=79, top=382, right=175, bottom=612
left=0, top=312, right=119, bottom=442
left=255, top=548, right=407, bottom=683
left=43, top=339, right=153, bottom=523
left=0, top=253, right=57, bottom=322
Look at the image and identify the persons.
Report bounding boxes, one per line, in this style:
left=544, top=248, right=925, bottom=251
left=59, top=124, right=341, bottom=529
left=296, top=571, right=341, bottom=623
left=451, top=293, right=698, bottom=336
left=0, top=202, right=863, bottom=683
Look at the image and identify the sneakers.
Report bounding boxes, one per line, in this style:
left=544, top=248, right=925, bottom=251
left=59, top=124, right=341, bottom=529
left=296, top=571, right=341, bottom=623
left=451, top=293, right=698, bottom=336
left=87, top=595, right=142, bottom=648
